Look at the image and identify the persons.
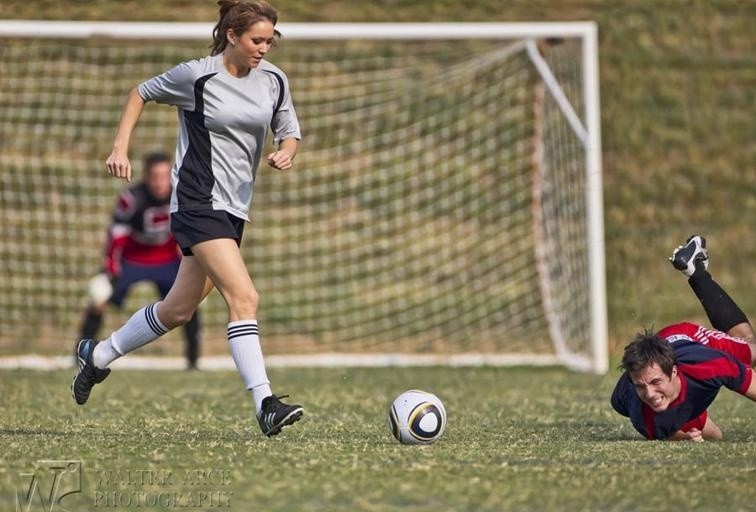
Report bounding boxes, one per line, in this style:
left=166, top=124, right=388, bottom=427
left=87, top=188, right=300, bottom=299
left=78, top=153, right=202, bottom=372
left=612, top=235, right=756, bottom=442
left=70, top=0, right=302, bottom=439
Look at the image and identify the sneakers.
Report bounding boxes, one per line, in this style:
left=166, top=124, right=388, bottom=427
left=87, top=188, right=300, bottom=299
left=666, top=231, right=712, bottom=280
left=68, top=336, right=112, bottom=406
left=254, top=389, right=305, bottom=439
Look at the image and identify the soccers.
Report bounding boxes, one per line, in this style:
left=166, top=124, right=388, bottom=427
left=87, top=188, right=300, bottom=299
left=388, top=389, right=447, bottom=444
